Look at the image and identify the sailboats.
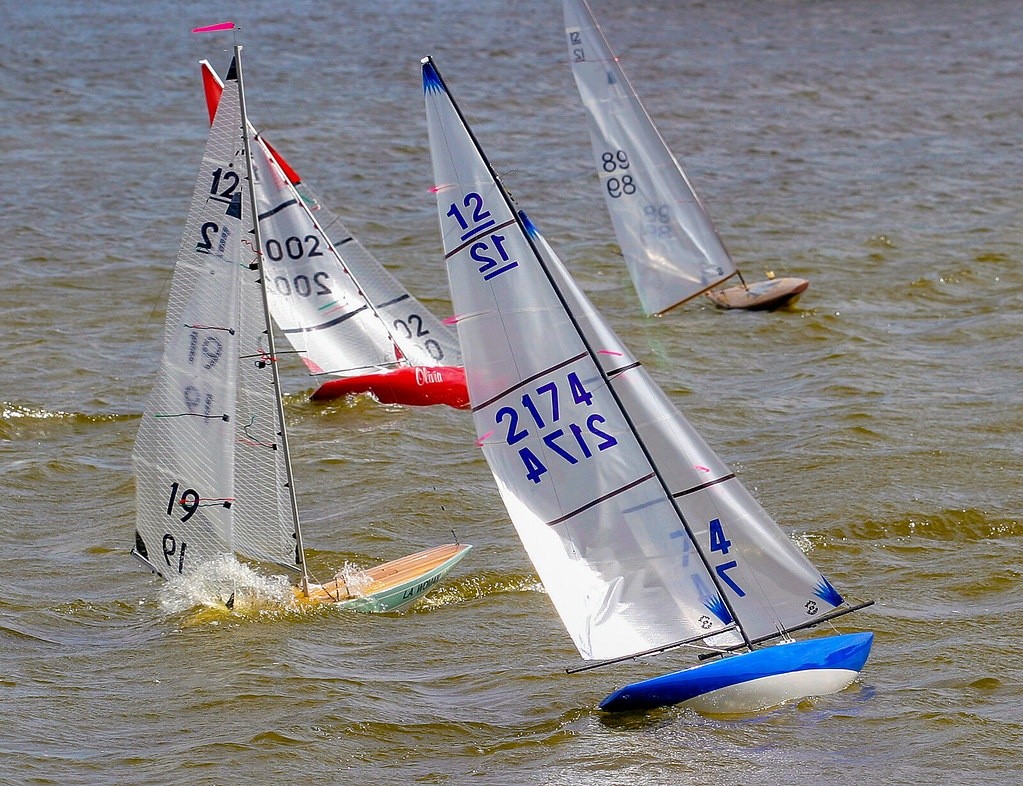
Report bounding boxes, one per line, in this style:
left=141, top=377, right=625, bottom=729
left=562, top=1, right=810, bottom=319
left=199, top=58, right=514, bottom=411
left=419, top=54, right=876, bottom=722
left=128, top=38, right=472, bottom=627
